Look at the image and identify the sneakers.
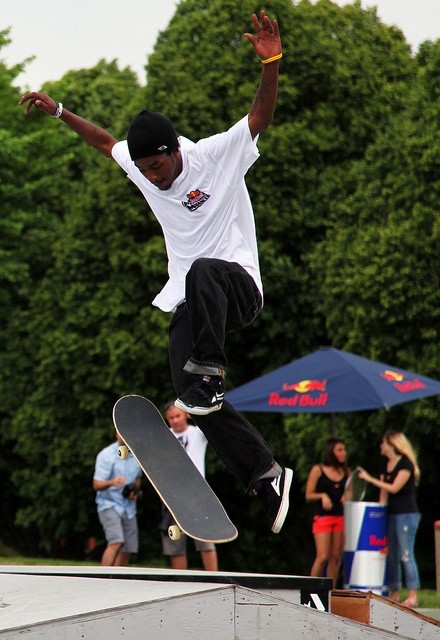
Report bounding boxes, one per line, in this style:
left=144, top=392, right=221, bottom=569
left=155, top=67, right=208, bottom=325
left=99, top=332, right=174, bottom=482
left=173, top=375, right=225, bottom=416
left=248, top=467, right=294, bottom=534
left=387, top=595, right=401, bottom=604
left=399, top=598, right=418, bottom=607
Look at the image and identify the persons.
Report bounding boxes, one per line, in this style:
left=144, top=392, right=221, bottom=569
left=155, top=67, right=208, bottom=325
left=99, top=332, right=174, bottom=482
left=355, top=429, right=423, bottom=610
left=304, top=437, right=356, bottom=588
left=17, top=9, right=294, bottom=536
left=159, top=398, right=219, bottom=571
left=92, top=425, right=140, bottom=567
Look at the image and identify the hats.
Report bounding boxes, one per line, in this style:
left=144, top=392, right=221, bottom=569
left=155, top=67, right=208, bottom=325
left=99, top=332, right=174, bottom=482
left=127, top=109, right=179, bottom=161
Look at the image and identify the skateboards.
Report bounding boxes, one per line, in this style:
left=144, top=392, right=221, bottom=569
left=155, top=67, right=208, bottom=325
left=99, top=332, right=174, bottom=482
left=113, top=393, right=238, bottom=544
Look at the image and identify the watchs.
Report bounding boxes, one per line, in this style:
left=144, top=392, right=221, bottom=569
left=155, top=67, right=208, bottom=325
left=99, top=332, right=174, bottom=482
left=52, top=100, right=66, bottom=122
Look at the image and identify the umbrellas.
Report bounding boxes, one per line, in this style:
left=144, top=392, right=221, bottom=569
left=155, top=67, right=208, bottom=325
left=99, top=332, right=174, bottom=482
left=225, top=344, right=439, bottom=445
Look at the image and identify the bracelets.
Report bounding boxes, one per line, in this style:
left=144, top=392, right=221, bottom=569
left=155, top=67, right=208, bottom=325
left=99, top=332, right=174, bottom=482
left=256, top=52, right=284, bottom=69
